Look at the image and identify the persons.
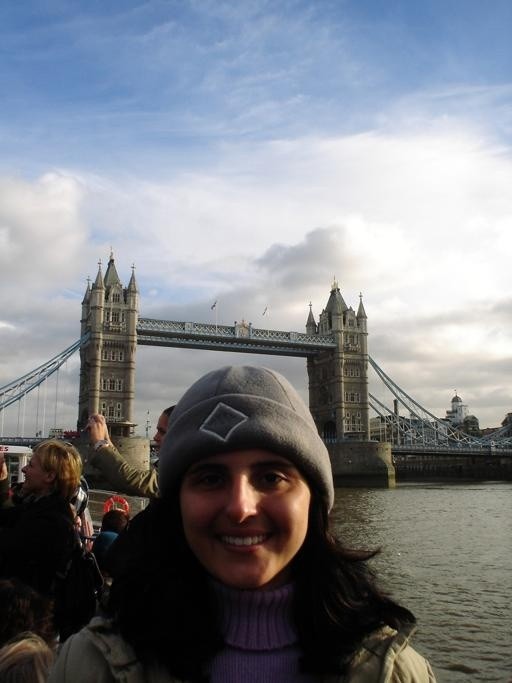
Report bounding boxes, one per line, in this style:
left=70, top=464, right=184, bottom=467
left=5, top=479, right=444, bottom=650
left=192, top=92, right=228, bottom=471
left=2, top=401, right=181, bottom=683
left=49, top=361, right=440, bottom=682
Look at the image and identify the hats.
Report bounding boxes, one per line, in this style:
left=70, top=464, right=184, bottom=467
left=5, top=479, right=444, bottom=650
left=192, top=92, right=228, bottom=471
left=157, top=365, right=335, bottom=516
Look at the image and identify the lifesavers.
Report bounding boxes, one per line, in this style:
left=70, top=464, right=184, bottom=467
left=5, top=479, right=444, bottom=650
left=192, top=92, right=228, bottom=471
left=104, top=496, right=129, bottom=514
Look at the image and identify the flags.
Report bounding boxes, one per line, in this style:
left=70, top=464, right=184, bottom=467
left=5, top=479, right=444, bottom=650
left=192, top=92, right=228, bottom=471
left=211, top=302, right=217, bottom=309
left=262, top=306, right=268, bottom=316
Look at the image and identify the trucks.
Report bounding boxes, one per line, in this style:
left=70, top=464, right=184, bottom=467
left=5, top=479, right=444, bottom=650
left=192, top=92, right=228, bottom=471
left=49, top=429, right=80, bottom=439
